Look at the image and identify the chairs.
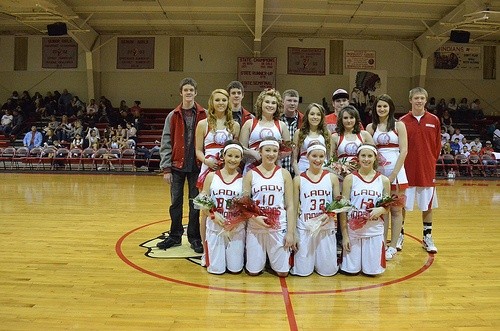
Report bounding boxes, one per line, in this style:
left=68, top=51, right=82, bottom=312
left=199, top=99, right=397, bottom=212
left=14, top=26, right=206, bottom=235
left=0, top=146, right=159, bottom=173
left=435, top=154, right=500, bottom=177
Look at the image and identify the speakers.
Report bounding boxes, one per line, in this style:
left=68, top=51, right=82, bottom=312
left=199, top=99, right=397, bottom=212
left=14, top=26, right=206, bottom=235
left=47, top=22, right=67, bottom=36
left=450, top=30, right=470, bottom=43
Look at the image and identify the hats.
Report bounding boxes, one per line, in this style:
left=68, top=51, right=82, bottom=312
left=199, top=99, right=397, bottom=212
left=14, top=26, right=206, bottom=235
left=332, top=89, right=349, bottom=101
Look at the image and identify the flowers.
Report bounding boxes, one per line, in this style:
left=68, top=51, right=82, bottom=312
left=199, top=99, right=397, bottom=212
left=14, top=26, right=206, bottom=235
left=279, top=141, right=295, bottom=153
left=324, top=192, right=409, bottom=226
left=191, top=190, right=240, bottom=212
left=324, top=159, right=348, bottom=176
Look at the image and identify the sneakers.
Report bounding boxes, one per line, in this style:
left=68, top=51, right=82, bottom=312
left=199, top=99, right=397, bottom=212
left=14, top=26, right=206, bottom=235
left=422, top=237, right=438, bottom=254
left=385, top=246, right=397, bottom=261
left=396, top=233, right=404, bottom=251
left=157, top=237, right=182, bottom=250
left=191, top=240, right=204, bottom=254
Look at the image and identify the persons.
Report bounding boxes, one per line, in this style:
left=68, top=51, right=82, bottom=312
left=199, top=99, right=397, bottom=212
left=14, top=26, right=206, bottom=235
left=0, top=88, right=144, bottom=169
left=159, top=77, right=500, bottom=277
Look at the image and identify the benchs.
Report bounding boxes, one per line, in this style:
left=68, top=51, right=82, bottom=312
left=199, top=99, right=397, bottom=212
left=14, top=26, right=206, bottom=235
left=452, top=119, right=484, bottom=143
left=0, top=107, right=174, bottom=147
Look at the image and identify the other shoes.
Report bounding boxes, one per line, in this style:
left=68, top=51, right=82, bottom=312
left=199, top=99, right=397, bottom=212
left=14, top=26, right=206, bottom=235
left=337, top=244, right=343, bottom=255
